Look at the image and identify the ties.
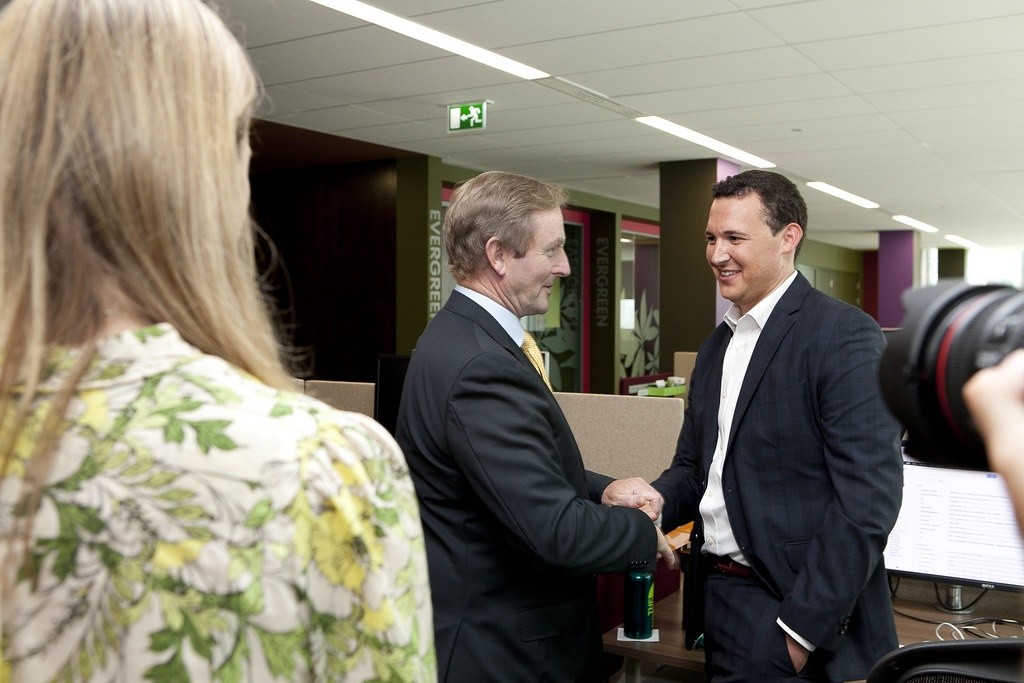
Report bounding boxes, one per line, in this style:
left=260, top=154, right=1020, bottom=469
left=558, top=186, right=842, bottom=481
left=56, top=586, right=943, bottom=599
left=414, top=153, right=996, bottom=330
left=520, top=331, right=553, bottom=395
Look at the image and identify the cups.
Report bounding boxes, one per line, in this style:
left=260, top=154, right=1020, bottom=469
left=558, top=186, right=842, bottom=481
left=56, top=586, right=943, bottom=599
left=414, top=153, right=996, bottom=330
left=668, top=376, right=685, bottom=385
left=655, top=380, right=666, bottom=388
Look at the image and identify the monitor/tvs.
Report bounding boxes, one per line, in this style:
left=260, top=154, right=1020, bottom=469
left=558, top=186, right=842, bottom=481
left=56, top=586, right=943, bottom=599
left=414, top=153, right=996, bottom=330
left=882, top=440, right=1024, bottom=614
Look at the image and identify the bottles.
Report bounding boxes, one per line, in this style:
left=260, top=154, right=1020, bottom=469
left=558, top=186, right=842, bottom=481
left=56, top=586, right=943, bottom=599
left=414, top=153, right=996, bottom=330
left=623, top=570, right=654, bottom=639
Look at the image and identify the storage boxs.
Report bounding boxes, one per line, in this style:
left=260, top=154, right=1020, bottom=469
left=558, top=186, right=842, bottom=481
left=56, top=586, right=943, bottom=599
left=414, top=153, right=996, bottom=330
left=648, top=384, right=686, bottom=396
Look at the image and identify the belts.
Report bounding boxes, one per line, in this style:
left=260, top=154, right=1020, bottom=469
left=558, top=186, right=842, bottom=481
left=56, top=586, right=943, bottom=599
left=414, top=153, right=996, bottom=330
left=708, top=554, right=754, bottom=578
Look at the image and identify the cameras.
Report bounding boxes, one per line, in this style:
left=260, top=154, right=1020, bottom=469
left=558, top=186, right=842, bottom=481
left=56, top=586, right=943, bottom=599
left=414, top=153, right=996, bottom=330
left=878, top=276, right=1024, bottom=472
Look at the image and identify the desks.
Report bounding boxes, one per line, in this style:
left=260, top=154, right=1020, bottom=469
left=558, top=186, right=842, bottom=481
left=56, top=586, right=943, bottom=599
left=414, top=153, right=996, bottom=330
left=604, top=576, right=1024, bottom=683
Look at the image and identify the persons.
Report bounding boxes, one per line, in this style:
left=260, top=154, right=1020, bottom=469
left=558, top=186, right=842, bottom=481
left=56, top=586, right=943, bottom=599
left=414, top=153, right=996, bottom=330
left=650, top=169, right=904, bottom=683
left=963, top=348, right=1024, bottom=545
left=0, top=0, right=439, bottom=682
left=393, top=170, right=675, bottom=683
